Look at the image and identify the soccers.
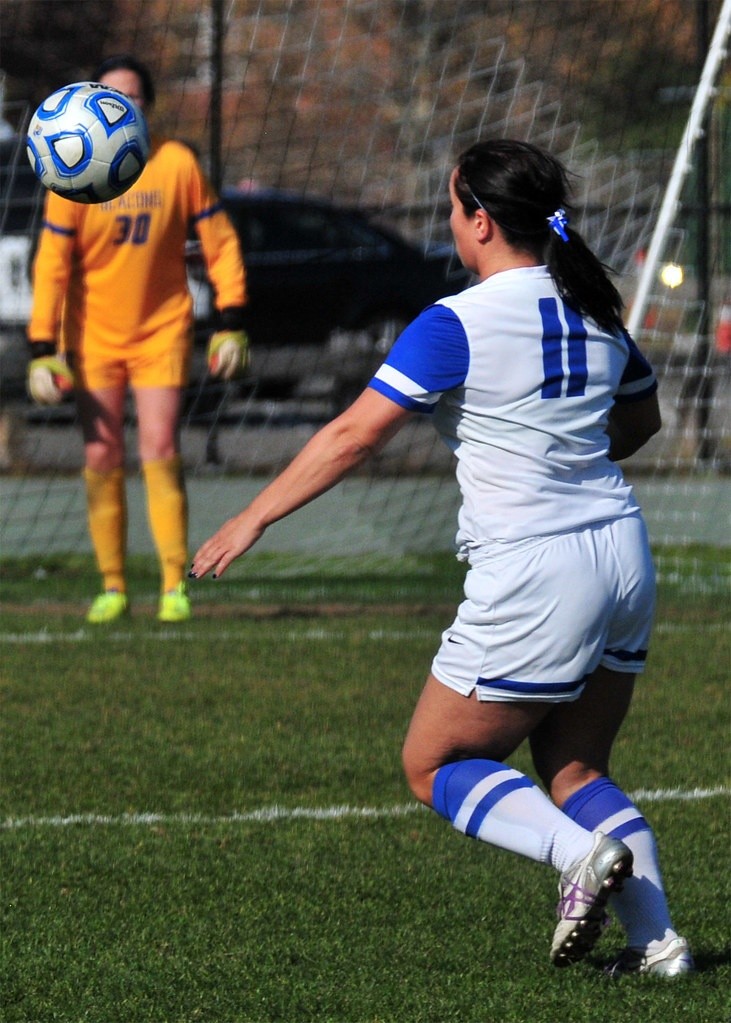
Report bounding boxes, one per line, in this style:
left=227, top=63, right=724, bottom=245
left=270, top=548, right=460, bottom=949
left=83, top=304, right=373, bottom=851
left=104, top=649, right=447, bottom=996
left=26, top=80, right=151, bottom=203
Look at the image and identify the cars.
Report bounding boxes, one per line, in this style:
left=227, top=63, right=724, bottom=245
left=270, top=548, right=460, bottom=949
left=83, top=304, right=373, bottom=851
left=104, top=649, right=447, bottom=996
left=191, top=191, right=470, bottom=341
left=1, top=131, right=213, bottom=376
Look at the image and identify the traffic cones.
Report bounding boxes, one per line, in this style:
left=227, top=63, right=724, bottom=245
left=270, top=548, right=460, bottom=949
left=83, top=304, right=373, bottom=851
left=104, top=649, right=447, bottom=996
left=710, top=298, right=731, bottom=356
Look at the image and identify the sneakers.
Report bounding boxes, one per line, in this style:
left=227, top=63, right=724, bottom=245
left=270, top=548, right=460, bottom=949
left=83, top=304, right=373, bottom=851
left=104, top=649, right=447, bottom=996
left=87, top=591, right=130, bottom=624
left=550, top=831, right=633, bottom=968
left=602, top=937, right=696, bottom=977
left=158, top=581, right=192, bottom=622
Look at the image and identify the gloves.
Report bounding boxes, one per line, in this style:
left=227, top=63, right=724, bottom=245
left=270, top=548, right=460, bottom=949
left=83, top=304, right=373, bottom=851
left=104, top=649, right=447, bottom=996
left=28, top=356, right=74, bottom=406
left=206, top=329, right=251, bottom=381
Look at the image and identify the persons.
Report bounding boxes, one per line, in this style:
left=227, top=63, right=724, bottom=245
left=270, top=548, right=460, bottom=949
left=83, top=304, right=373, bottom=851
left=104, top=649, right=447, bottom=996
left=21, top=54, right=251, bottom=624
left=188, top=136, right=695, bottom=980
